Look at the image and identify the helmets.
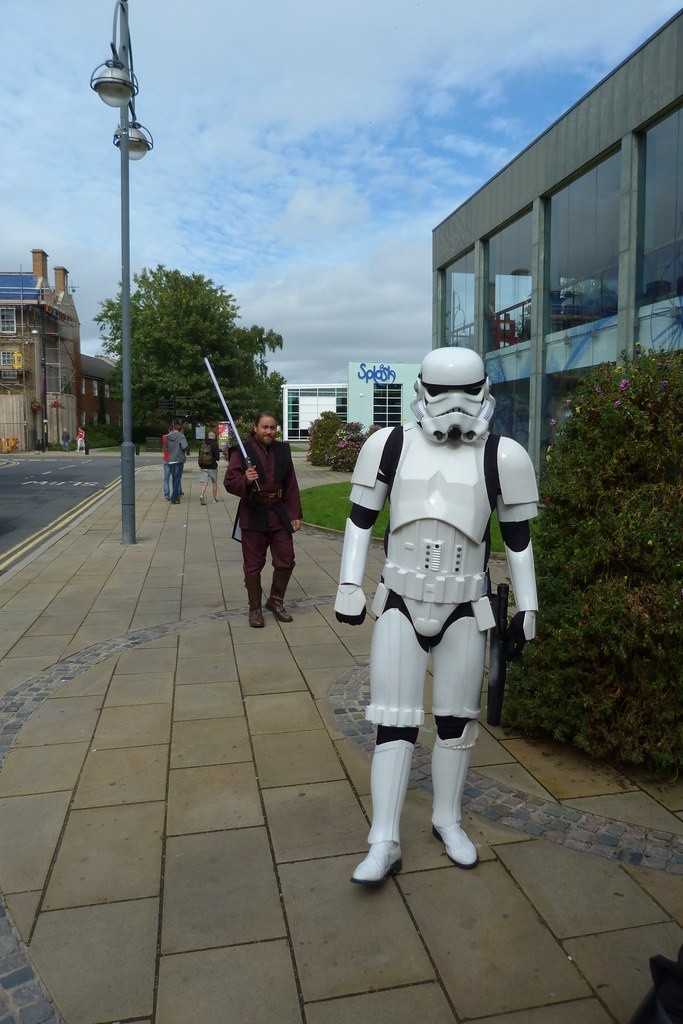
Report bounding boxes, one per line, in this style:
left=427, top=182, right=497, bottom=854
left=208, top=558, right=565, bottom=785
left=410, top=347, right=496, bottom=442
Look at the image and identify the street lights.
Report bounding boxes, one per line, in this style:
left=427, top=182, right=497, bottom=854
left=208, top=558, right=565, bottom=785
left=31, top=329, right=47, bottom=454
left=90, top=0, right=153, bottom=545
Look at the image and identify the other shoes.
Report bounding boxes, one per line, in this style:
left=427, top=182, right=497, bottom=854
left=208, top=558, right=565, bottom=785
left=170, top=497, right=181, bottom=504
left=83, top=451, right=85, bottom=453
left=164, top=495, right=170, bottom=501
left=212, top=498, right=217, bottom=503
left=200, top=495, right=206, bottom=505
left=180, top=492, right=185, bottom=498
left=76, top=450, right=80, bottom=452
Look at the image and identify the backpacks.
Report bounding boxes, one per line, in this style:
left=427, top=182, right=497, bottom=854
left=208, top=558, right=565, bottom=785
left=198, top=441, right=216, bottom=465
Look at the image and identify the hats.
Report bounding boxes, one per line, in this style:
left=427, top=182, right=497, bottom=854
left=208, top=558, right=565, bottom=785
left=207, top=432, right=216, bottom=440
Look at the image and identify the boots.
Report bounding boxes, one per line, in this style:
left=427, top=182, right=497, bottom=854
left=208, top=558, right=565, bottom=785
left=265, top=562, right=295, bottom=621
left=351, top=737, right=414, bottom=884
left=429, top=719, right=480, bottom=869
left=243, top=573, right=265, bottom=626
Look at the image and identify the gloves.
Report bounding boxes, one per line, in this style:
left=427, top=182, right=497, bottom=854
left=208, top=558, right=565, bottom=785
left=506, top=611, right=528, bottom=654
left=335, top=603, right=368, bottom=627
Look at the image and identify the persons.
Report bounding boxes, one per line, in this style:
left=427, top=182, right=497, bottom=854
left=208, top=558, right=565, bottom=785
left=198, top=432, right=221, bottom=505
left=224, top=412, right=304, bottom=628
left=75, top=426, right=85, bottom=452
left=166, top=425, right=190, bottom=504
left=61, top=427, right=70, bottom=451
left=333, top=346, right=539, bottom=884
left=162, top=428, right=185, bottom=500
